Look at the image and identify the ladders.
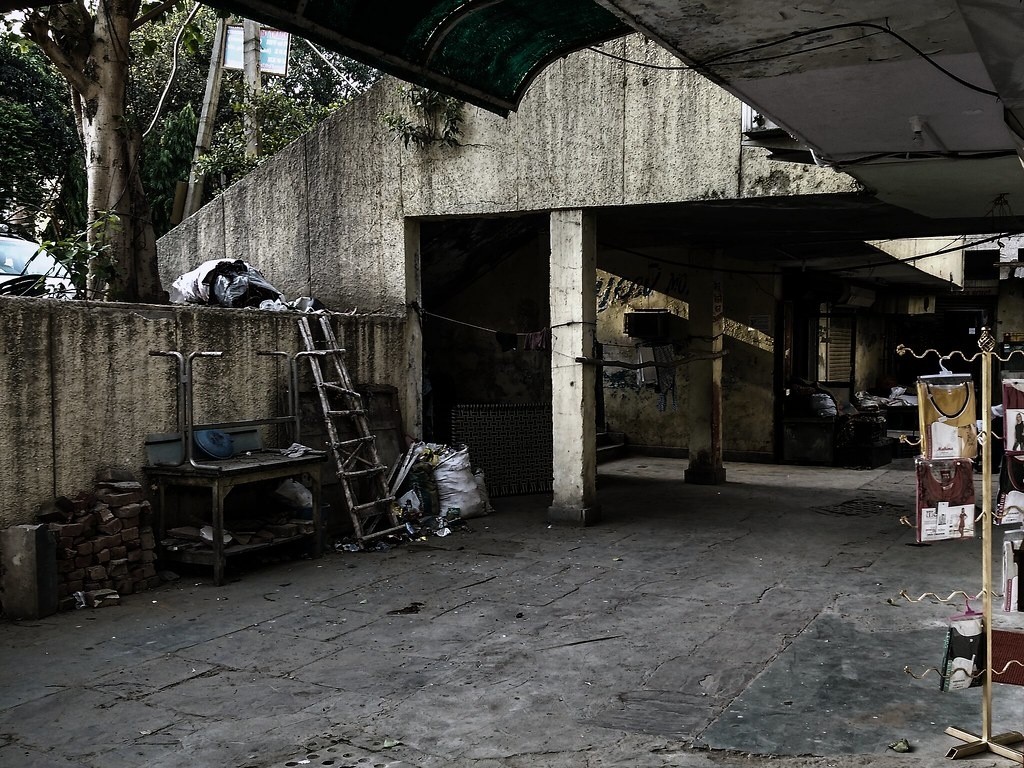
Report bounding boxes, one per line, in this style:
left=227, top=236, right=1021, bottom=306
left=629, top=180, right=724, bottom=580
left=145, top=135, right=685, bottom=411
left=297, top=316, right=408, bottom=550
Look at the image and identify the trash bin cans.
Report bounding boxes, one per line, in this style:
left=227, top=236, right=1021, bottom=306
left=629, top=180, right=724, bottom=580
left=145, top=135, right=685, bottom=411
left=783, top=416, right=835, bottom=461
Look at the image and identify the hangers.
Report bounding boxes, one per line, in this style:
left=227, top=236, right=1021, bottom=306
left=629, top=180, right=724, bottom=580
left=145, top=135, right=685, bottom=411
left=1005, top=517, right=1024, bottom=534
left=918, top=356, right=971, bottom=379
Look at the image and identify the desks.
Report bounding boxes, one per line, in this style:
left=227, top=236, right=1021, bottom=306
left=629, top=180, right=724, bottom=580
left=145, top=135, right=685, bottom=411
left=141, top=450, right=328, bottom=587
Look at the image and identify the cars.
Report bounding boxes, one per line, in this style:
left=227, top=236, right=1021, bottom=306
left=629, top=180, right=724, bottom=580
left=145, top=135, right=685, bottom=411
left=0, top=237, right=85, bottom=301
